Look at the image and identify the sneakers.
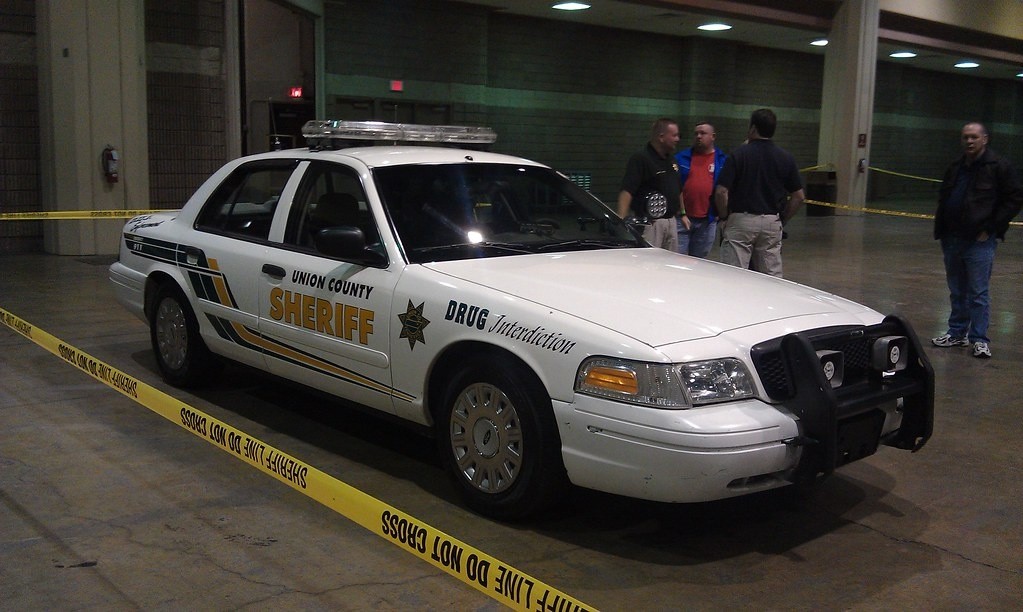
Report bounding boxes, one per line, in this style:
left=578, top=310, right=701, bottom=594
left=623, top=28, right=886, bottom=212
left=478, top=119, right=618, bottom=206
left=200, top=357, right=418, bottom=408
left=931, top=331, right=970, bottom=346
left=973, top=341, right=992, bottom=357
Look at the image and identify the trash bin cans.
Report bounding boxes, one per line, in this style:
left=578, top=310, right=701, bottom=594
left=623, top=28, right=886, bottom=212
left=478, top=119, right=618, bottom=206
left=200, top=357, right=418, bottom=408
left=805, top=171, right=837, bottom=217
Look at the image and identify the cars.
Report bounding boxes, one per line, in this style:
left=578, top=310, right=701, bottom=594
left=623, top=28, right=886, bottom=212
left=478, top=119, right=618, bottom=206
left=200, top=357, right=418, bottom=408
left=107, top=119, right=935, bottom=523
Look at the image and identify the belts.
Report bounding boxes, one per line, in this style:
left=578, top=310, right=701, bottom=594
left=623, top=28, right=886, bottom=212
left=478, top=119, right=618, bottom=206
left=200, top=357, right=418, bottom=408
left=731, top=208, right=778, bottom=215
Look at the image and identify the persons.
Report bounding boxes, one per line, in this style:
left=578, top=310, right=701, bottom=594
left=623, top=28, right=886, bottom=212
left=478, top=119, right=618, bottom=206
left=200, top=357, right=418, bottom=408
left=674, top=121, right=727, bottom=260
left=931, top=123, right=1023, bottom=358
left=715, top=108, right=804, bottom=278
left=616, top=118, right=692, bottom=253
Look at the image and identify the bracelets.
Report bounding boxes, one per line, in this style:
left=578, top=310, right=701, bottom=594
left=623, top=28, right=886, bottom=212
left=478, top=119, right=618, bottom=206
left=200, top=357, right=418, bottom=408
left=680, top=209, right=686, bottom=216
left=719, top=216, right=727, bottom=221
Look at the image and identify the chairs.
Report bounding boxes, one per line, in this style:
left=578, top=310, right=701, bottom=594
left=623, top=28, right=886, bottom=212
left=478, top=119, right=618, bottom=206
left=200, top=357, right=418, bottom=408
left=314, top=194, right=366, bottom=260
